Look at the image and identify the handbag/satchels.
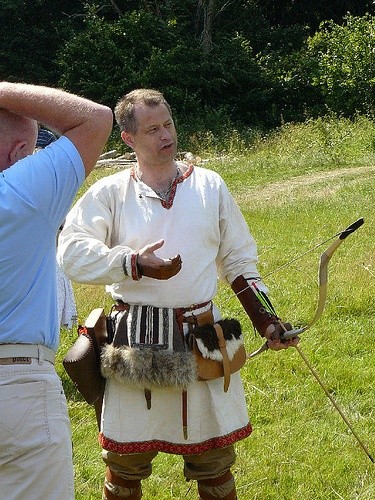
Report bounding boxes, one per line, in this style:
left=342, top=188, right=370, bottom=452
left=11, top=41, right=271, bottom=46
left=186, top=320, right=246, bottom=392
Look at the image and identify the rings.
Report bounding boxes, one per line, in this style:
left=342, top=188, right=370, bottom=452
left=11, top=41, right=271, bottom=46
left=163, top=258, right=172, bottom=266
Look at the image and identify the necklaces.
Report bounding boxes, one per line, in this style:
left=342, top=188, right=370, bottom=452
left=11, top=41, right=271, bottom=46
left=134, top=161, right=181, bottom=203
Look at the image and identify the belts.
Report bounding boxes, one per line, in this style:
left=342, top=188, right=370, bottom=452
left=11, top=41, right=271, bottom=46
left=0, top=344, right=55, bottom=365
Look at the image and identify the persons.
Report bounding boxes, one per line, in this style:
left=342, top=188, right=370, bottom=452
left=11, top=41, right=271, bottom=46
left=1, top=82, right=113, bottom=500
left=55, top=88, right=301, bottom=500
